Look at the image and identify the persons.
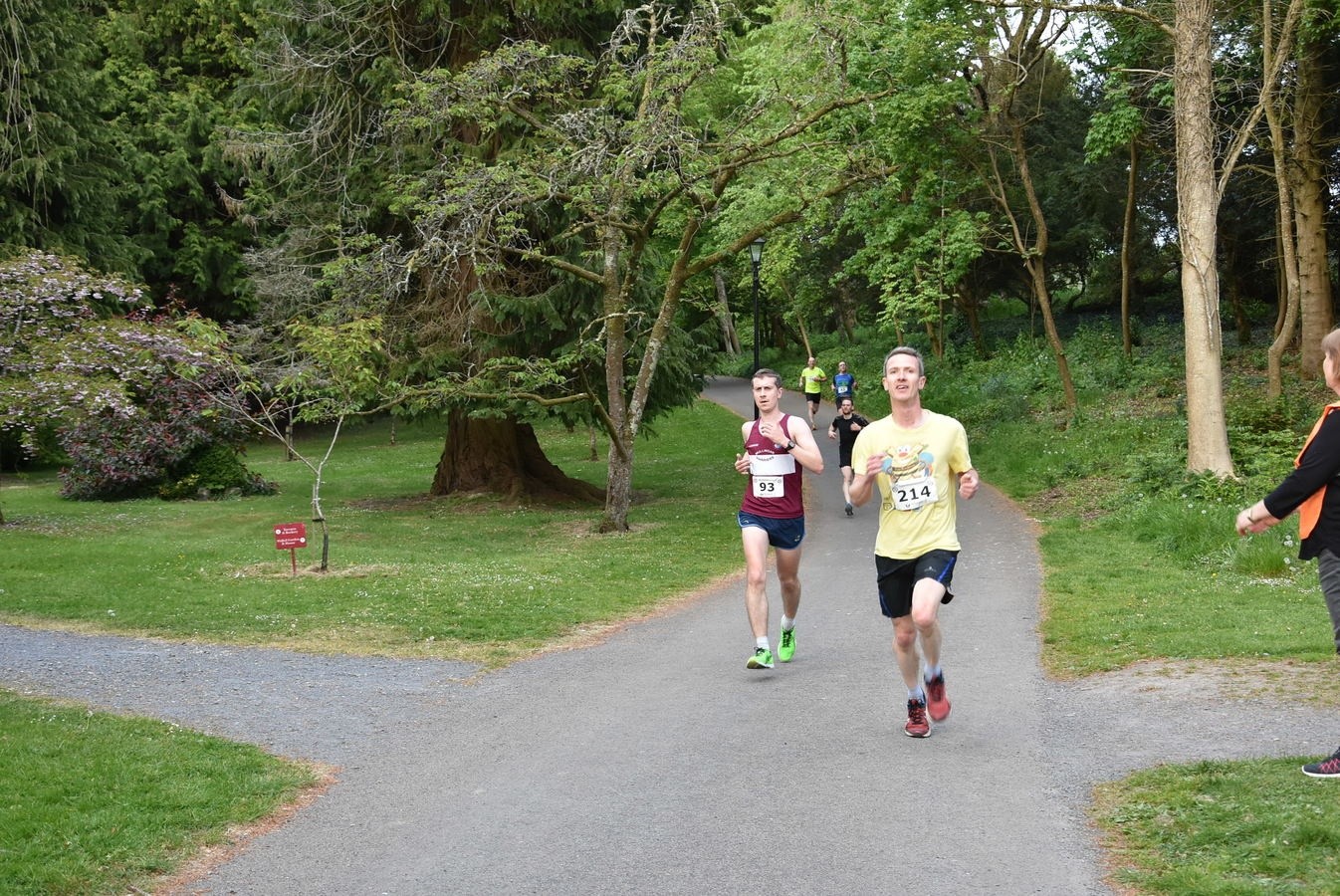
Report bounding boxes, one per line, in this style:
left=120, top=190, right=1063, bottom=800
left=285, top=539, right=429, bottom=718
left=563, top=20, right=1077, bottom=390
left=799, top=357, right=827, bottom=431
left=1234, top=328, right=1340, bottom=778
left=828, top=398, right=869, bottom=517
left=831, top=361, right=858, bottom=413
left=848, top=346, right=979, bottom=738
left=734, top=368, right=825, bottom=669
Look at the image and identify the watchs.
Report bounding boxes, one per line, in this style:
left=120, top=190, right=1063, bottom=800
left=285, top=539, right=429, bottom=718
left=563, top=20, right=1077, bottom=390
left=784, top=440, right=796, bottom=451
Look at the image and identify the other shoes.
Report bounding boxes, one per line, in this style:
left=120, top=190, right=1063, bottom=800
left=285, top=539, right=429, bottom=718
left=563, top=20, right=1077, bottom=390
left=845, top=504, right=853, bottom=517
left=812, top=425, right=817, bottom=431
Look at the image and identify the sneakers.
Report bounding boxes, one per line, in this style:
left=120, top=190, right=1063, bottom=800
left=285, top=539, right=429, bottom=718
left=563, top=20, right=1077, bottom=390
left=906, top=696, right=931, bottom=738
left=777, top=620, right=796, bottom=663
left=924, top=670, right=950, bottom=723
left=746, top=647, right=774, bottom=669
left=1302, top=745, right=1340, bottom=779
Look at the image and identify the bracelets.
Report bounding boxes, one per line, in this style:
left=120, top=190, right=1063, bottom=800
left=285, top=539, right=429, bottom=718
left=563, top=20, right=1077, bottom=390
left=1246, top=507, right=1255, bottom=523
left=861, top=426, right=863, bottom=430
left=819, top=379, right=820, bottom=382
left=1263, top=516, right=1273, bottom=527
